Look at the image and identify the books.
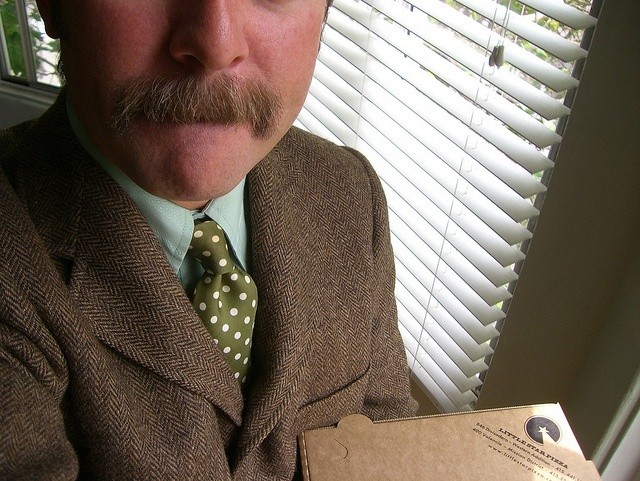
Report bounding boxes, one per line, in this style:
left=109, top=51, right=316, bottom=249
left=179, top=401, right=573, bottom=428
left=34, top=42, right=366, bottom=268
left=298, top=402, right=603, bottom=481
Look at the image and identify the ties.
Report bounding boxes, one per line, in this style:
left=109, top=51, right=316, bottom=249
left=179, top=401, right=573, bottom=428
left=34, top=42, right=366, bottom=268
left=188, top=218, right=260, bottom=391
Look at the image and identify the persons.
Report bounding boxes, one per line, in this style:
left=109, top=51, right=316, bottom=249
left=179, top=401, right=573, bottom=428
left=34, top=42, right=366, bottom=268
left=0, top=0, right=420, bottom=481
